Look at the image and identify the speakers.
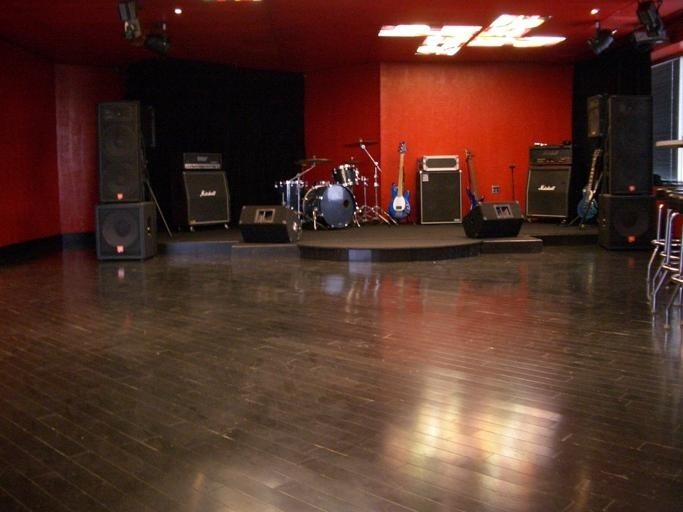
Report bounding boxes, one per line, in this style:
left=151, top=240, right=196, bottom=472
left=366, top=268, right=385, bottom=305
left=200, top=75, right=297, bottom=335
left=419, top=170, right=463, bottom=225
left=597, top=195, right=655, bottom=251
left=181, top=171, right=231, bottom=226
left=97, top=202, right=158, bottom=259
left=462, top=202, right=525, bottom=240
left=526, top=165, right=572, bottom=218
left=97, top=100, right=145, bottom=203
left=239, top=204, right=302, bottom=243
left=604, top=96, right=654, bottom=195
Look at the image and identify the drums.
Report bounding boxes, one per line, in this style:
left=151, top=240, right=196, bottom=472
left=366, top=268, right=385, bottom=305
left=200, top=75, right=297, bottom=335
left=332, top=164, right=359, bottom=187
left=276, top=180, right=307, bottom=214
left=303, top=185, right=355, bottom=229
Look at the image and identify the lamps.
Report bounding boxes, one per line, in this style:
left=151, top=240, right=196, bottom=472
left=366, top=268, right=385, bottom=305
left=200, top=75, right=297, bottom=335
left=119, top=0, right=141, bottom=40
left=146, top=35, right=172, bottom=55
left=587, top=30, right=614, bottom=55
left=636, top=0, right=660, bottom=32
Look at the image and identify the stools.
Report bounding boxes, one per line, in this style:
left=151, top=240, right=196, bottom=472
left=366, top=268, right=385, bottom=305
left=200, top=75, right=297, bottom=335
left=647, top=188, right=683, bottom=331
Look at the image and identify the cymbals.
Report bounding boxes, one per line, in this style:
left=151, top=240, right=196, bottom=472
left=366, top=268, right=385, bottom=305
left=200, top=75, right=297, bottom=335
left=345, top=142, right=378, bottom=146
left=297, top=159, right=332, bottom=164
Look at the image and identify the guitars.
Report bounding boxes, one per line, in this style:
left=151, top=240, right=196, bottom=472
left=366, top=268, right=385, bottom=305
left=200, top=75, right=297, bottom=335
left=465, top=149, right=484, bottom=210
left=389, top=141, right=411, bottom=219
left=577, top=149, right=599, bottom=221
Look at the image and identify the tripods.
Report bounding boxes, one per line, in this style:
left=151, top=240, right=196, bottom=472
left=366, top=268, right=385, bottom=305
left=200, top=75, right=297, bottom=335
left=347, top=145, right=399, bottom=227
left=290, top=162, right=327, bottom=231
